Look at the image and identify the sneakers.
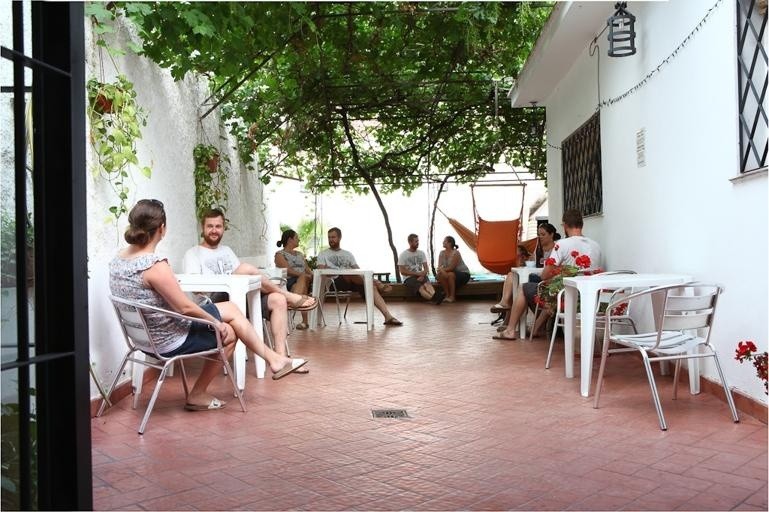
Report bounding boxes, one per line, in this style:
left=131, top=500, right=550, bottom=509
left=443, top=297, right=456, bottom=303
left=432, top=293, right=447, bottom=302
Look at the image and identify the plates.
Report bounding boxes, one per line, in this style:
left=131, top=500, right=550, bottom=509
left=525, top=266, right=536, bottom=269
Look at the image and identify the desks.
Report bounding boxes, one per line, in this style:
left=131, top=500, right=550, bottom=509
left=563, top=274, right=701, bottom=397
left=309, top=268, right=374, bottom=331
left=131, top=274, right=266, bottom=398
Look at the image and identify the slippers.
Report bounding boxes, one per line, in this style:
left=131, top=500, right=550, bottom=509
left=272, top=358, right=307, bottom=383
left=383, top=317, right=403, bottom=327
left=290, top=367, right=310, bottom=374
left=525, top=328, right=539, bottom=339
left=491, top=304, right=512, bottom=310
left=378, top=284, right=392, bottom=294
left=297, top=323, right=309, bottom=329
left=184, top=397, right=227, bottom=412
left=493, top=330, right=518, bottom=340
left=287, top=292, right=318, bottom=310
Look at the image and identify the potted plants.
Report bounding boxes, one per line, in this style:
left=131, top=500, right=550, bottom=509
left=86, top=74, right=151, bottom=247
left=193, top=144, right=240, bottom=238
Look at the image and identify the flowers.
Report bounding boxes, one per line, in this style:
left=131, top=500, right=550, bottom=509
left=735, top=341, right=768, bottom=396
left=533, top=251, right=629, bottom=322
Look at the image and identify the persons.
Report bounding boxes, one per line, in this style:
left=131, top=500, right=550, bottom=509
left=274, top=229, right=328, bottom=329
left=490, top=222, right=561, bottom=332
left=107, top=198, right=309, bottom=412
left=181, top=208, right=318, bottom=375
left=492, top=208, right=601, bottom=340
left=316, top=227, right=403, bottom=326
left=397, top=234, right=446, bottom=305
left=437, top=235, right=471, bottom=304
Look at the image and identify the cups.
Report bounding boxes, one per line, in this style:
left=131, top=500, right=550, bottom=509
left=525, top=261, right=536, bottom=267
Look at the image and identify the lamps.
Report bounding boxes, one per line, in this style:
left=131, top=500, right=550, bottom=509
left=607, top=1, right=636, bottom=57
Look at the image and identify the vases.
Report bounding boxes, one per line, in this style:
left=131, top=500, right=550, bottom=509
left=561, top=312, right=613, bottom=357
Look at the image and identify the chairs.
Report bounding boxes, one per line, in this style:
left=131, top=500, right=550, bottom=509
left=545, top=270, right=637, bottom=369
left=593, top=281, right=740, bottom=431
left=96, top=295, right=246, bottom=434
left=313, top=268, right=353, bottom=325
left=530, top=267, right=595, bottom=344
